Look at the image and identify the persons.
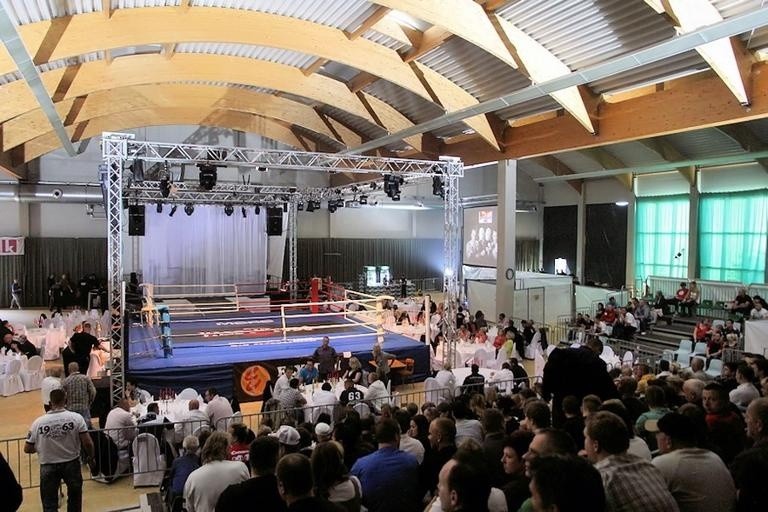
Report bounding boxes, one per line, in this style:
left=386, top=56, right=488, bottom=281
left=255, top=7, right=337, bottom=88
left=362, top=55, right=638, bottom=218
left=0, top=452, right=24, bottom=511
left=1, top=320, right=38, bottom=359
left=24, top=362, right=96, bottom=512
left=161, top=330, right=768, bottom=512
left=567, top=280, right=699, bottom=346
left=383, top=274, right=536, bottom=361
left=61, top=320, right=111, bottom=379
left=11, top=273, right=108, bottom=326
left=102, top=378, right=235, bottom=476
left=693, top=286, right=768, bottom=370
left=284, top=274, right=367, bottom=312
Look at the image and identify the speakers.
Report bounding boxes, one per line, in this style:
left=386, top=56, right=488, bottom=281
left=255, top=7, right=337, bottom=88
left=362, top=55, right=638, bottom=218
left=267, top=208, right=282, bottom=236
left=127, top=207, right=145, bottom=236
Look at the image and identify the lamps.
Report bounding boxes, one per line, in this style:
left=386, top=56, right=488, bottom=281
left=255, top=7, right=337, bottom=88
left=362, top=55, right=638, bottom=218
left=103, top=141, right=448, bottom=221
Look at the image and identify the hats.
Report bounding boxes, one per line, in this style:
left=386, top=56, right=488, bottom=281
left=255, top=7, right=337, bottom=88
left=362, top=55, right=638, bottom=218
left=315, top=422, right=332, bottom=436
left=268, top=425, right=301, bottom=445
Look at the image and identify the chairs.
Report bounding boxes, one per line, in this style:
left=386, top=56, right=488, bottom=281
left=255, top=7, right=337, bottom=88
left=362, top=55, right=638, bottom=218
left=1, top=296, right=637, bottom=509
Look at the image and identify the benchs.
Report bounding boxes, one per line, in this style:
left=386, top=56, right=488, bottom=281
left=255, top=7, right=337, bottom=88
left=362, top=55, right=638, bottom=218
left=637, top=295, right=765, bottom=509
